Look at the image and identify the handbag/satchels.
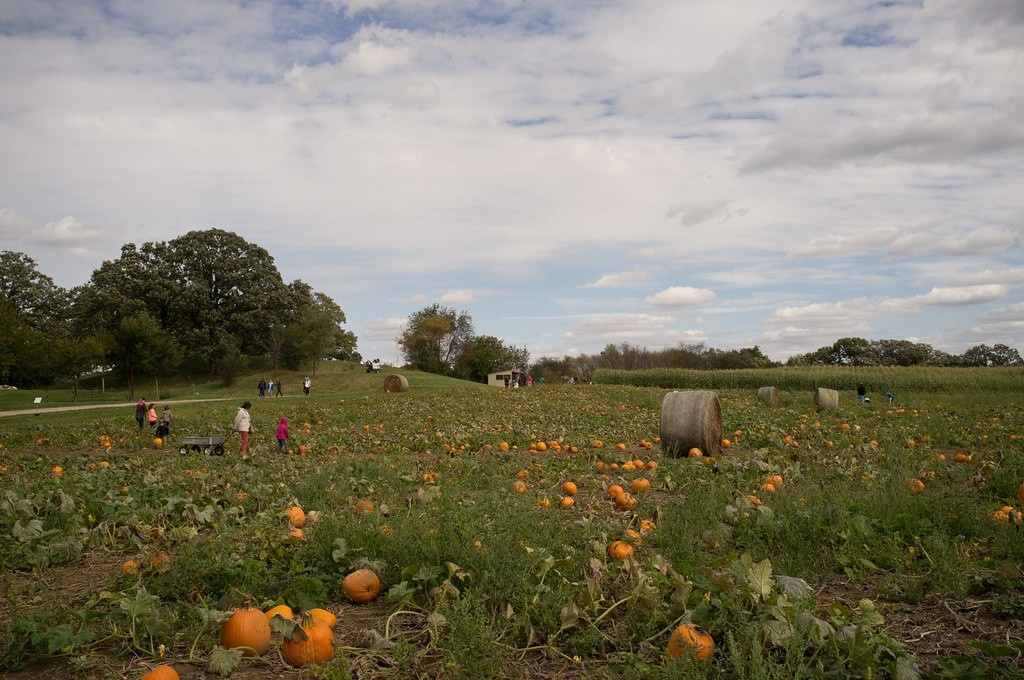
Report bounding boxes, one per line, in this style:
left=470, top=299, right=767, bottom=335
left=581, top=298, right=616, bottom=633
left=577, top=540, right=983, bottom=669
left=248, top=425, right=254, bottom=434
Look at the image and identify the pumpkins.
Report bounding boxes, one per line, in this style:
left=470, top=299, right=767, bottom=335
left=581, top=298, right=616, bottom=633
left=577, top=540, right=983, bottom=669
left=0, top=409, right=1024, bottom=680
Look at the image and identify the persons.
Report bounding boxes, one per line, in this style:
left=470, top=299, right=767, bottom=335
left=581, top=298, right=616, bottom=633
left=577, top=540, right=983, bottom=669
left=276, top=417, right=290, bottom=454
left=540, top=376, right=545, bottom=384
left=234, top=401, right=254, bottom=455
left=258, top=378, right=282, bottom=398
left=561, top=374, right=575, bottom=386
left=303, top=376, right=312, bottom=395
left=884, top=392, right=894, bottom=404
left=366, top=359, right=381, bottom=374
left=155, top=417, right=169, bottom=445
left=135, top=397, right=173, bottom=430
left=504, top=373, right=533, bottom=389
left=857, top=382, right=871, bottom=403
left=590, top=381, right=593, bottom=385
